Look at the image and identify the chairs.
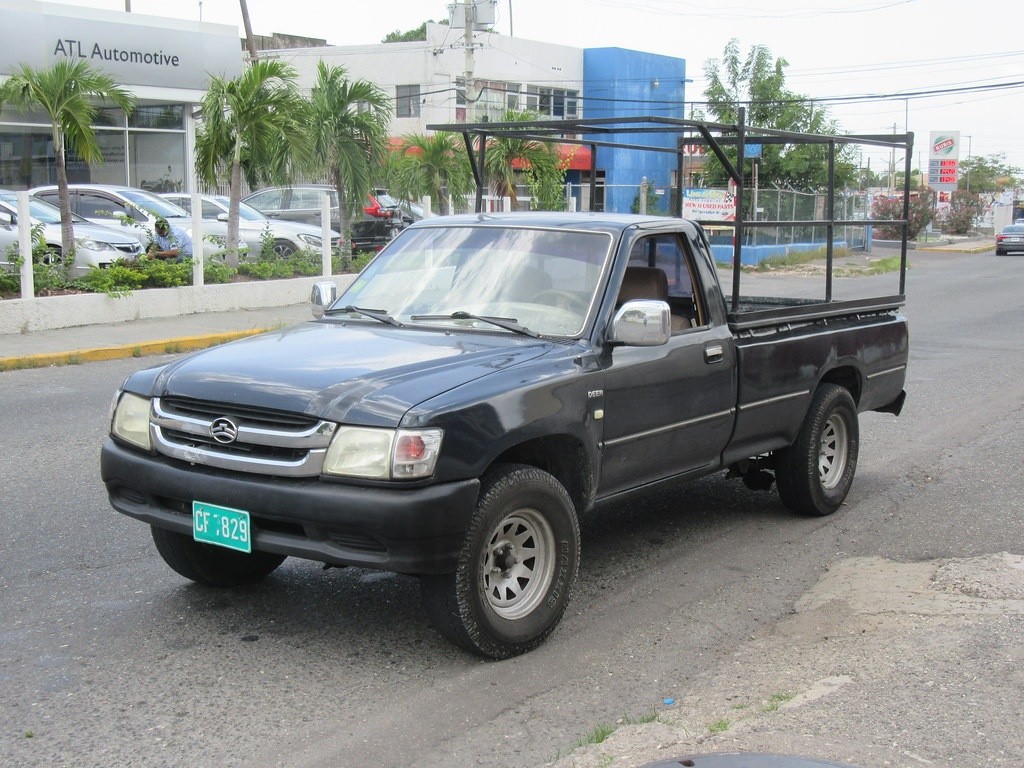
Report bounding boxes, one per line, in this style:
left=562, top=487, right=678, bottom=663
left=618, top=267, right=689, bottom=331
left=507, top=265, right=555, bottom=305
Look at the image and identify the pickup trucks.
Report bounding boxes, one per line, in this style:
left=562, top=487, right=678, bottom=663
left=102, top=212, right=912, bottom=657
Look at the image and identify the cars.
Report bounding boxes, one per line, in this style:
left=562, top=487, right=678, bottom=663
left=994, top=225, right=1024, bottom=255
left=394, top=196, right=443, bottom=224
left=1015, top=219, right=1024, bottom=225
left=0, top=188, right=146, bottom=282
left=162, top=193, right=342, bottom=260
left=23, top=183, right=248, bottom=268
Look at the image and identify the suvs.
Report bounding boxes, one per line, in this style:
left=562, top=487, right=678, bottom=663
left=242, top=184, right=414, bottom=252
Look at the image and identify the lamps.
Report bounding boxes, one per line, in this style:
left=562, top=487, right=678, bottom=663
left=650, top=78, right=660, bottom=88
